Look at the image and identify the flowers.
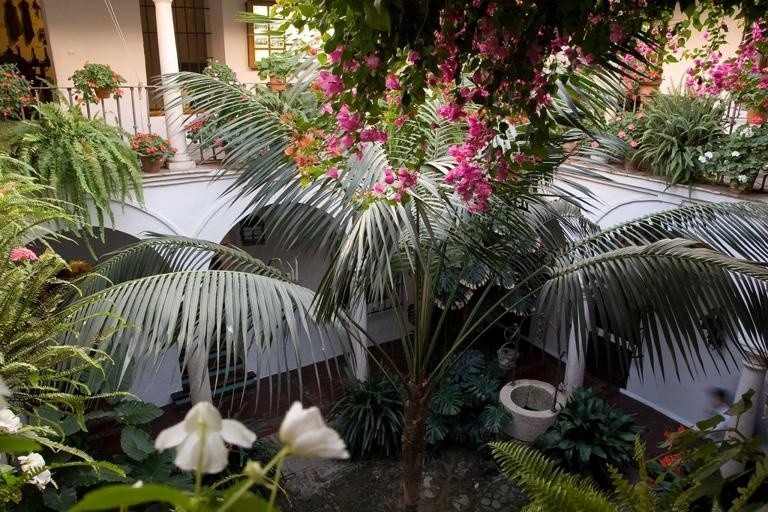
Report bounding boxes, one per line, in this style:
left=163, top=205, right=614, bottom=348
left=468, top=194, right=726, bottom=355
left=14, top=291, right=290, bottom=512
left=0, top=62, right=38, bottom=118
left=131, top=133, right=177, bottom=164
left=699, top=124, right=768, bottom=185
left=67, top=64, right=128, bottom=104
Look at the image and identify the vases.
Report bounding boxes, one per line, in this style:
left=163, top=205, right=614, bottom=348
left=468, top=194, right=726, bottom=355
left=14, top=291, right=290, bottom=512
left=726, top=168, right=755, bottom=195
left=140, top=158, right=164, bottom=173
left=94, top=87, right=112, bottom=98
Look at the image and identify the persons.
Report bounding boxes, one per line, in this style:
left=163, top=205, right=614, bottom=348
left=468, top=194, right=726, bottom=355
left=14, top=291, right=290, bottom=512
left=702, top=386, right=732, bottom=442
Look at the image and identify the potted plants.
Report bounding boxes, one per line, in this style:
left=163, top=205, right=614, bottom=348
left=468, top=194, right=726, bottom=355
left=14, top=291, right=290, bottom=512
left=255, top=51, right=298, bottom=92
